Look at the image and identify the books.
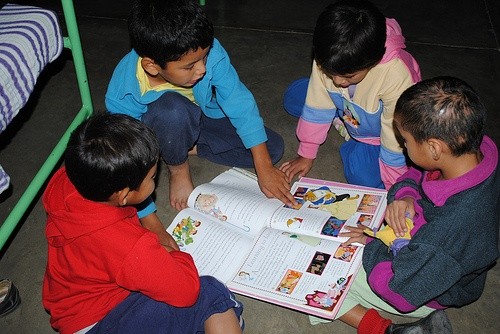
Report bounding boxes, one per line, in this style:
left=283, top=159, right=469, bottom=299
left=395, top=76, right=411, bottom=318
left=165, top=166, right=388, bottom=322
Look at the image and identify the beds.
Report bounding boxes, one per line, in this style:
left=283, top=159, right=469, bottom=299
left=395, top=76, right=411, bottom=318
left=0, top=0, right=96, bottom=250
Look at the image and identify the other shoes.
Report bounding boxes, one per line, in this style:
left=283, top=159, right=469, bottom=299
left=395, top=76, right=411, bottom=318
left=0, top=279, right=21, bottom=317
left=390, top=310, right=452, bottom=334
left=333, top=117, right=351, bottom=142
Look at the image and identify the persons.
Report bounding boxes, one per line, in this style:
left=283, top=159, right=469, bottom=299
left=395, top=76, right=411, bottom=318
left=309, top=76, right=500, bottom=334
left=279, top=0, right=422, bottom=190
left=41, top=113, right=245, bottom=334
left=104, top=0, right=298, bottom=210
